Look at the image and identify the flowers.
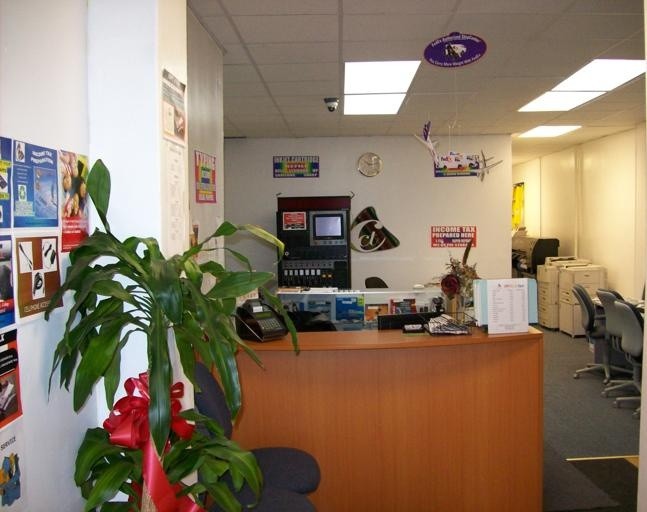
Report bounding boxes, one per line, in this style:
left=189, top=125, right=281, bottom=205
left=423, top=238, right=479, bottom=307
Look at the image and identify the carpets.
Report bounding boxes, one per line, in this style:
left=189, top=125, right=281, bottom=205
left=566, top=455, right=639, bottom=512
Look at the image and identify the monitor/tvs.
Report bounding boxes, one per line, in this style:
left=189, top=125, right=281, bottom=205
left=309, top=211, right=348, bottom=246
left=377, top=312, right=442, bottom=329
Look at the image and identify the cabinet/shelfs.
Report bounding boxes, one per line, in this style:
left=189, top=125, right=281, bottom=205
left=276, top=289, right=442, bottom=323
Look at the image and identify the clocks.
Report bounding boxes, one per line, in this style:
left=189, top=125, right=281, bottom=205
left=358, top=152, right=383, bottom=176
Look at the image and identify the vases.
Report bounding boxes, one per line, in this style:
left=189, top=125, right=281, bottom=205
left=447, top=294, right=464, bottom=322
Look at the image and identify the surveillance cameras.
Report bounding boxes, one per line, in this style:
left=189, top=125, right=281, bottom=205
left=324, top=97, right=339, bottom=112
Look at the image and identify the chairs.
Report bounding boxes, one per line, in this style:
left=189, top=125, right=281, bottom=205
left=194, top=361, right=320, bottom=512
left=571, top=283, right=644, bottom=419
